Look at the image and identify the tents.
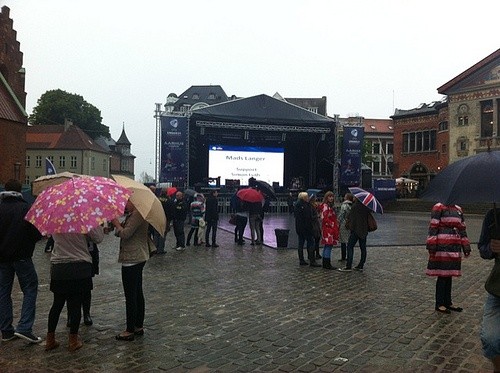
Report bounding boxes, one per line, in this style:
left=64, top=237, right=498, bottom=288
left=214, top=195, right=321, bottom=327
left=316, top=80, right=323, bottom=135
left=190, top=93, right=339, bottom=203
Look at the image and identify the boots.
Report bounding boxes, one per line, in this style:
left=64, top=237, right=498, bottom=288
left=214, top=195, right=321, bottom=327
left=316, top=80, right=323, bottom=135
left=45, top=332, right=58, bottom=351
left=68, top=333, right=82, bottom=351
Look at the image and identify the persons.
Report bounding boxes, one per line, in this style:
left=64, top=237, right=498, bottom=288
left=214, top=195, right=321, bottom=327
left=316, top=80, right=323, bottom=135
left=45, top=237, right=55, bottom=253
left=396, top=179, right=416, bottom=199
left=44, top=225, right=104, bottom=351
left=111, top=198, right=150, bottom=342
left=294, top=191, right=371, bottom=271
left=0, top=178, right=43, bottom=343
left=287, top=192, right=294, bottom=214
left=229, top=177, right=271, bottom=245
left=148, top=185, right=219, bottom=255
left=67, top=228, right=109, bottom=328
left=426, top=202, right=471, bottom=313
left=478, top=209, right=500, bottom=373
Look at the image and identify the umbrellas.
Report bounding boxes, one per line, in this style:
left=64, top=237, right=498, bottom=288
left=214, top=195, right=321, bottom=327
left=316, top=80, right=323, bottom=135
left=396, top=177, right=419, bottom=183
left=426, top=144, right=500, bottom=240
left=112, top=175, right=166, bottom=237
left=348, top=187, right=383, bottom=215
left=24, top=175, right=134, bottom=237
left=253, top=180, right=276, bottom=200
left=237, top=188, right=263, bottom=203
left=185, top=189, right=195, bottom=196
left=31, top=171, right=76, bottom=197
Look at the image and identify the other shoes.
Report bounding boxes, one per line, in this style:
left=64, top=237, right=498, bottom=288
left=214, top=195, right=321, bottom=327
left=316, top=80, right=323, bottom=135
left=448, top=304, right=463, bottom=311
left=436, top=306, right=451, bottom=313
left=15, top=331, right=42, bottom=343
left=115, top=333, right=134, bottom=341
left=67, top=317, right=71, bottom=327
left=84, top=313, right=93, bottom=325
left=159, top=240, right=219, bottom=254
left=135, top=330, right=144, bottom=335
left=1, top=332, right=15, bottom=341
left=299, top=254, right=364, bottom=272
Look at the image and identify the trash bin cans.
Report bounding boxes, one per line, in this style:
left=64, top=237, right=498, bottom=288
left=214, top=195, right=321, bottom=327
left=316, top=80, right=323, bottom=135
left=274, top=228, right=289, bottom=248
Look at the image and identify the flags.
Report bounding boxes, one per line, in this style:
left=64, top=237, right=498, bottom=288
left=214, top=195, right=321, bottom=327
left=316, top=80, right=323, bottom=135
left=45, top=158, right=57, bottom=175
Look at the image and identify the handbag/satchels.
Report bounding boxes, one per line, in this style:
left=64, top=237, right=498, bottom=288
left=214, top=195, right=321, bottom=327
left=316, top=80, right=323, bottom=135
left=147, top=238, right=157, bottom=252
left=367, top=214, right=377, bottom=232
left=229, top=215, right=239, bottom=225
